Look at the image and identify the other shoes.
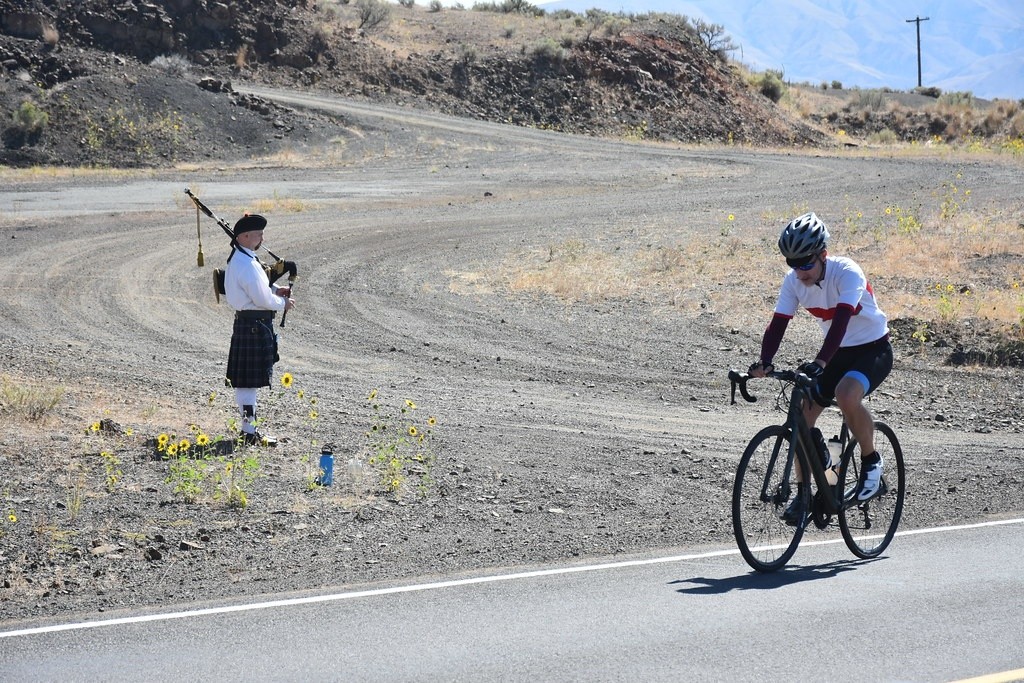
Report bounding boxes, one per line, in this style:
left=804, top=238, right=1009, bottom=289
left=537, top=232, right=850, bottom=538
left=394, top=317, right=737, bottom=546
left=239, top=430, right=278, bottom=447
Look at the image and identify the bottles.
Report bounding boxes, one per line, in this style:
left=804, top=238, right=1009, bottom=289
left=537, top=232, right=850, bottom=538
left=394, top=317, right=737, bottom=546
left=319, top=445, right=334, bottom=486
left=824, top=435, right=842, bottom=486
left=810, top=427, right=832, bottom=470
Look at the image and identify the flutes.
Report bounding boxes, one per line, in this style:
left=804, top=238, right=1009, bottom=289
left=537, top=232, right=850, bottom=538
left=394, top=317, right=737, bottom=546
left=183, top=187, right=298, bottom=327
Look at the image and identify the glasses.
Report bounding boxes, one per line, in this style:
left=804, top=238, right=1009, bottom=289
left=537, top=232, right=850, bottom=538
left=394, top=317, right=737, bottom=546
left=791, top=254, right=820, bottom=272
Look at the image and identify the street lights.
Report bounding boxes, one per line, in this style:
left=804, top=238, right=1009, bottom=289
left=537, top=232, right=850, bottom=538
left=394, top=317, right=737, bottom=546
left=904, top=15, right=932, bottom=89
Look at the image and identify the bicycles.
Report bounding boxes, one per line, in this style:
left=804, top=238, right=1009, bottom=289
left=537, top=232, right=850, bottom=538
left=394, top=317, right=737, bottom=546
left=725, top=359, right=907, bottom=571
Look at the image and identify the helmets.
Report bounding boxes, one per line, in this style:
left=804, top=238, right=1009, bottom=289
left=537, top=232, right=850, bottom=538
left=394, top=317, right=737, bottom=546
left=777, top=212, right=830, bottom=259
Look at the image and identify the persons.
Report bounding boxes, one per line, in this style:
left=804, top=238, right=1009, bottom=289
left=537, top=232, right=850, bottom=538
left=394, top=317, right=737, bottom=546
left=225, top=214, right=295, bottom=447
left=748, top=211, right=893, bottom=522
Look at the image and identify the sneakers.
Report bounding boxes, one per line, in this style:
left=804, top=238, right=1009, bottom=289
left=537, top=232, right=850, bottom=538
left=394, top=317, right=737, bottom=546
left=854, top=451, right=886, bottom=501
left=784, top=493, right=809, bottom=519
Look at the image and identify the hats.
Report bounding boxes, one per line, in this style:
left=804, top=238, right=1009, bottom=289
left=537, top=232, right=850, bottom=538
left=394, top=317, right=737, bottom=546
left=786, top=254, right=814, bottom=267
left=233, top=214, right=267, bottom=235
